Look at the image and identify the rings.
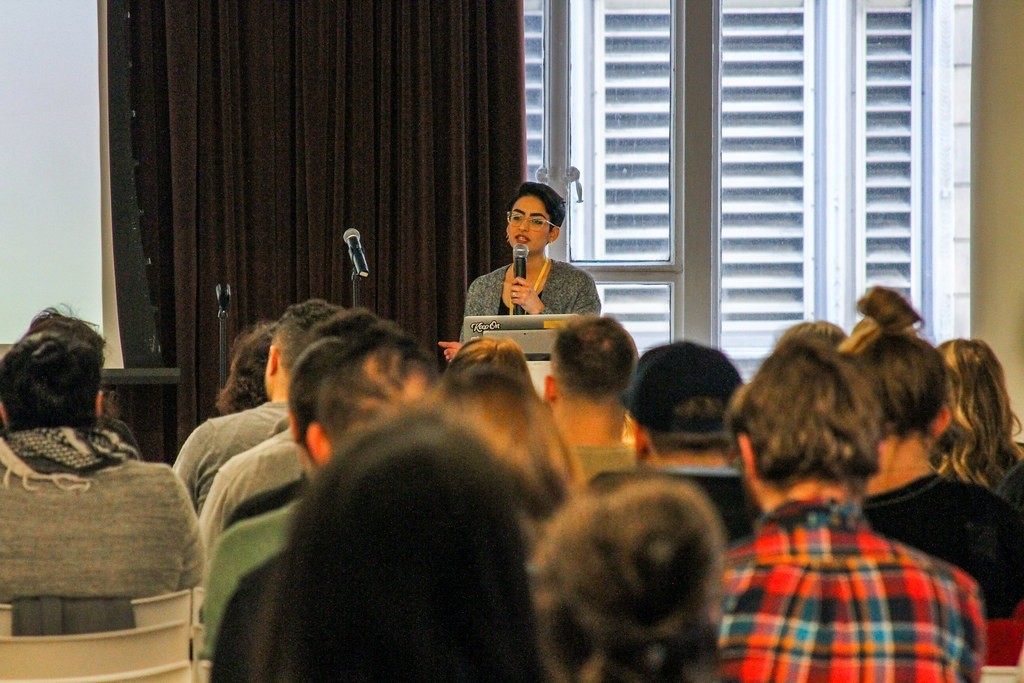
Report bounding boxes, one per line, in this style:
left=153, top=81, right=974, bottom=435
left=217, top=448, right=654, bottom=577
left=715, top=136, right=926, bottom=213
left=513, top=292, right=516, bottom=298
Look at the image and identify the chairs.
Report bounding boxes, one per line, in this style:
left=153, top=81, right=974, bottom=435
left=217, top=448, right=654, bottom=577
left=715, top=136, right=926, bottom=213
left=0, top=585, right=208, bottom=683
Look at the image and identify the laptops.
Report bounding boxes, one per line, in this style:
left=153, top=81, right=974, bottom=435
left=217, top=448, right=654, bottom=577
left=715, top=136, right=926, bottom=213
left=463, top=313, right=580, bottom=362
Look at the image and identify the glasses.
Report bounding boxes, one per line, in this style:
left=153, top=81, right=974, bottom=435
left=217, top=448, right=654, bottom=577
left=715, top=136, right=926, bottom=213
left=506, top=211, right=563, bottom=231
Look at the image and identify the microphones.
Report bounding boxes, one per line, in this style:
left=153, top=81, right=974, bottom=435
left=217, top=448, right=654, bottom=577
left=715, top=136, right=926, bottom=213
left=513, top=243, right=529, bottom=316
left=343, top=228, right=369, bottom=278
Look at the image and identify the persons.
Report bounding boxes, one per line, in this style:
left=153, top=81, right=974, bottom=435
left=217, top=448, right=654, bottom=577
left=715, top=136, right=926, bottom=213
left=439, top=181, right=602, bottom=363
left=0, top=284, right=1024, bottom=683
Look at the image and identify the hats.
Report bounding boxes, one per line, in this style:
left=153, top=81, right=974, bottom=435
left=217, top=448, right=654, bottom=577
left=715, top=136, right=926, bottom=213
left=622, top=342, right=745, bottom=429
